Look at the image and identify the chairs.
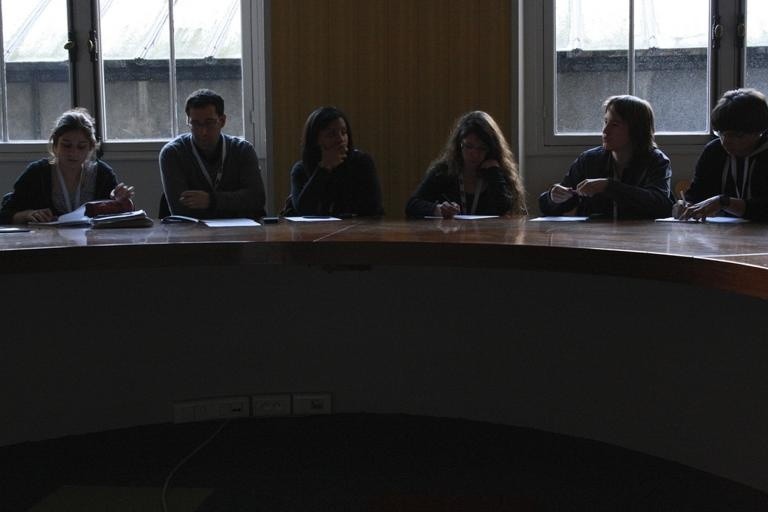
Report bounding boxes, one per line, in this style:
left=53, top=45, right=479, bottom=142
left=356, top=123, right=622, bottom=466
left=158, top=193, right=268, bottom=219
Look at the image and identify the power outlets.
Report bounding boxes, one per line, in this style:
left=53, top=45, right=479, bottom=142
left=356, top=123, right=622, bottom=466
left=173, top=394, right=250, bottom=423
left=251, top=391, right=293, bottom=418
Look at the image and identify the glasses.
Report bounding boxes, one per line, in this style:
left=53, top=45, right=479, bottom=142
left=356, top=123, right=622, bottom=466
left=459, top=140, right=489, bottom=153
left=188, top=117, right=223, bottom=130
left=713, top=128, right=753, bottom=142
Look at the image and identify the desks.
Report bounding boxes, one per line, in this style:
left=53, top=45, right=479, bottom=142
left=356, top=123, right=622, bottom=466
left=0, top=216, right=768, bottom=493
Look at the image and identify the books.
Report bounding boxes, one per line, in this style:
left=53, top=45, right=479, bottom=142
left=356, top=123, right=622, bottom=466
left=26, top=206, right=92, bottom=229
left=89, top=208, right=154, bottom=229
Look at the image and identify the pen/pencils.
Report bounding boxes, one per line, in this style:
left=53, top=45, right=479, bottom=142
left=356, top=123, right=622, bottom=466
left=571, top=190, right=577, bottom=192
left=441, top=193, right=461, bottom=215
left=680, top=190, right=687, bottom=205
left=303, top=216, right=330, bottom=218
left=179, top=196, right=184, bottom=201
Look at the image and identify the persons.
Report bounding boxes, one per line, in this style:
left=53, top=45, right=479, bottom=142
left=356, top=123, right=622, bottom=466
left=538, top=95, right=676, bottom=221
left=671, top=88, right=767, bottom=225
left=1, top=108, right=136, bottom=224
left=402, top=110, right=528, bottom=220
left=160, top=89, right=269, bottom=219
left=278, top=104, right=387, bottom=216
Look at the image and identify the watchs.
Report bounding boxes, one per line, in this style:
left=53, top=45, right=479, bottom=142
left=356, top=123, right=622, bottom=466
left=718, top=192, right=731, bottom=212
